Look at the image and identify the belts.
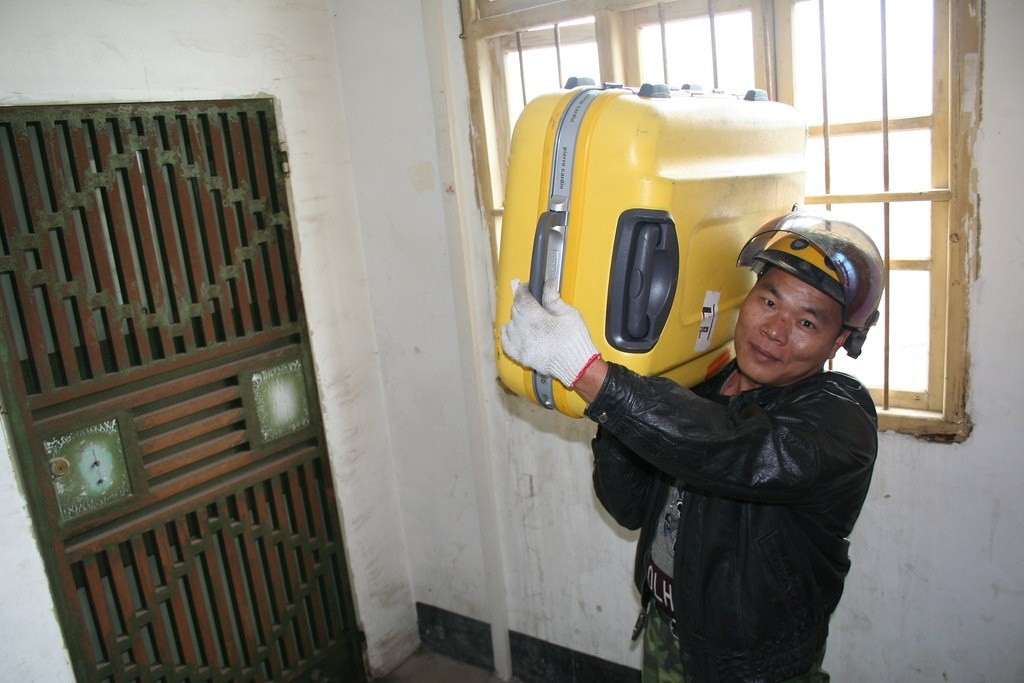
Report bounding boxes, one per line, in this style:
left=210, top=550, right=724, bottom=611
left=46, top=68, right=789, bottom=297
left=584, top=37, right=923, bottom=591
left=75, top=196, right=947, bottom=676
left=652, top=597, right=680, bottom=638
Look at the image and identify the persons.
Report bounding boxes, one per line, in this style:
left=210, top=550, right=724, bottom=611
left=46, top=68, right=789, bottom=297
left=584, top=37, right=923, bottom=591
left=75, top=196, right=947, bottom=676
left=500, top=214, right=886, bottom=683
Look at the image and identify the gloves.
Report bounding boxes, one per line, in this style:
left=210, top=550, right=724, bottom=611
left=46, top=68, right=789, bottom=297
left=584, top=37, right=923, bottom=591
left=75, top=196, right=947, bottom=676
left=500, top=281, right=601, bottom=392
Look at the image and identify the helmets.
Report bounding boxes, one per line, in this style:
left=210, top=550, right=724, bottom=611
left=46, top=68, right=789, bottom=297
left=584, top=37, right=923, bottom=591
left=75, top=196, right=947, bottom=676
left=736, top=213, right=888, bottom=363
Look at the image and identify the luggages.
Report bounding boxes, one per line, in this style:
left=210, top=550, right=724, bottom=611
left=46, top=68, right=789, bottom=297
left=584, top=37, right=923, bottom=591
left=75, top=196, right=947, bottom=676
left=493, top=77, right=809, bottom=419
